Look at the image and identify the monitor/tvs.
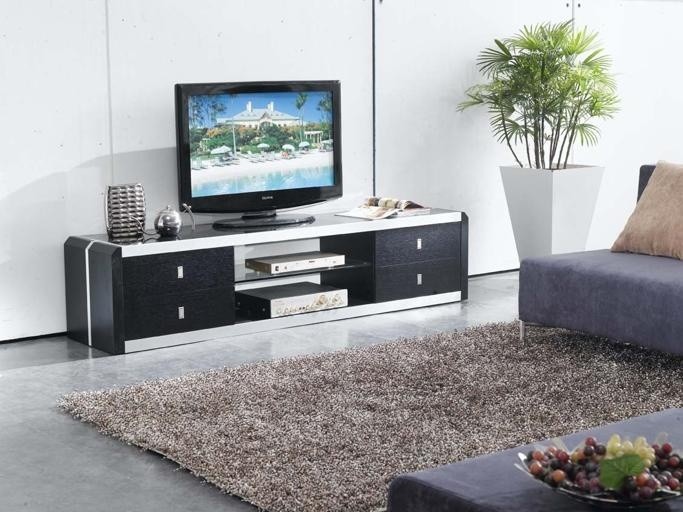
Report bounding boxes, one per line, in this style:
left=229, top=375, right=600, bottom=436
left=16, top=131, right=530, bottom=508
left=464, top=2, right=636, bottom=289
left=175, top=79, right=343, bottom=228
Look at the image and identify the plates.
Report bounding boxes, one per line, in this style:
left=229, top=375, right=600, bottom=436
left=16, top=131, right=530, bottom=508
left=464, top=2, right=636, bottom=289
left=513, top=429, right=683, bottom=510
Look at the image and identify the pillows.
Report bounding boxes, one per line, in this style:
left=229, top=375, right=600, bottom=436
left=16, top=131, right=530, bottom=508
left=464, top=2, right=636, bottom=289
left=611, top=160, right=683, bottom=261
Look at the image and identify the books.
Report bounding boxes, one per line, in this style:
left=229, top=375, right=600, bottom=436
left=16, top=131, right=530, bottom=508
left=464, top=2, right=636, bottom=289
left=334, top=197, right=425, bottom=221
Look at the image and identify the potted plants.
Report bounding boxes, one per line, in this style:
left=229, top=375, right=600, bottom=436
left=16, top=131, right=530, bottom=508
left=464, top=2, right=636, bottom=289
left=455, top=17, right=621, bottom=263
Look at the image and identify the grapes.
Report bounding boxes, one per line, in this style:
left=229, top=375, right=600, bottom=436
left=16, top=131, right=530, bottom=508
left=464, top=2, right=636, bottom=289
left=527, top=434, right=683, bottom=501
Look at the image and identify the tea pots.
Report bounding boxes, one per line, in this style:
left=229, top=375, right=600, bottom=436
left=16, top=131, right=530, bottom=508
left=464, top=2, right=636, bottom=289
left=155, top=202, right=196, bottom=239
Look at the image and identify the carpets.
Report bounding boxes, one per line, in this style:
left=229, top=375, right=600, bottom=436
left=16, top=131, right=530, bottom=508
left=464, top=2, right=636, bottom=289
left=56, top=319, right=683, bottom=512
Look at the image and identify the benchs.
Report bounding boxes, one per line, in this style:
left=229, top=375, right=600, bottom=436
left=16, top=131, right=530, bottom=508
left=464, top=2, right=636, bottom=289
left=518, top=164, right=683, bottom=356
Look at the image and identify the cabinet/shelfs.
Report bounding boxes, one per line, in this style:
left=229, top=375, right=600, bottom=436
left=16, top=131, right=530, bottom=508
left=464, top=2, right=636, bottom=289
left=63, top=207, right=469, bottom=356
